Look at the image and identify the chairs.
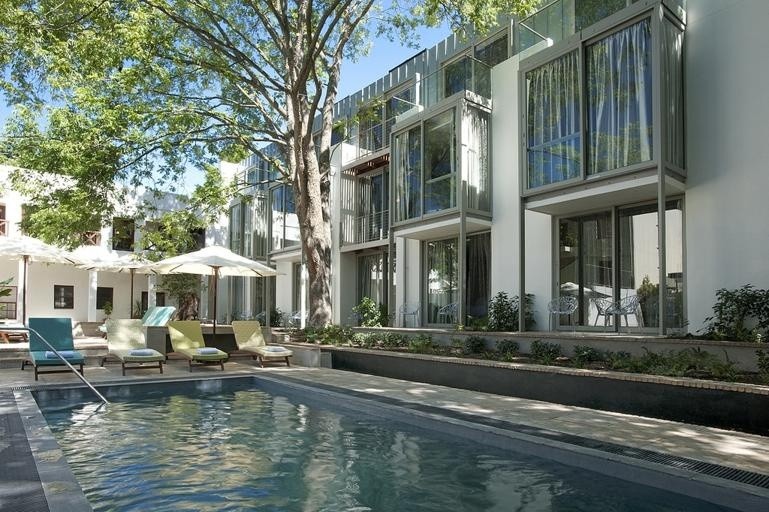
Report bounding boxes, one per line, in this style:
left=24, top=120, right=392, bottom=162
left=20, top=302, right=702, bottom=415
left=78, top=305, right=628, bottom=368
left=438, top=302, right=458, bottom=328
left=604, top=295, right=646, bottom=336
left=546, top=296, right=578, bottom=332
left=399, top=302, right=423, bottom=328
left=97, top=305, right=176, bottom=339
left=100, top=318, right=294, bottom=376
left=20, top=317, right=85, bottom=382
left=591, top=297, right=629, bottom=327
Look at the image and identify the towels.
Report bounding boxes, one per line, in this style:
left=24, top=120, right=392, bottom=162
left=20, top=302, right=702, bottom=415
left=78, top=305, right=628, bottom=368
left=45, top=351, right=74, bottom=358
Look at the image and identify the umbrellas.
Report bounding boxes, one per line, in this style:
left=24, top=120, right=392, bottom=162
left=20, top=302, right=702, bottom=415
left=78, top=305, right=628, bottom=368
left=138, top=244, right=288, bottom=339
left=0, top=234, right=82, bottom=326
left=77, top=252, right=156, bottom=318
